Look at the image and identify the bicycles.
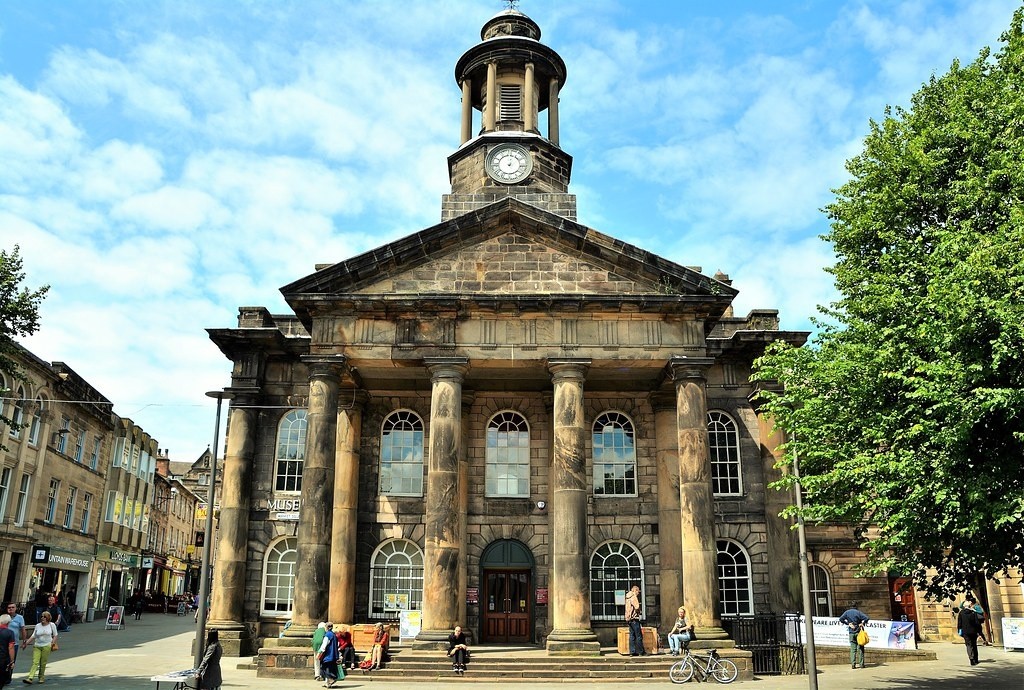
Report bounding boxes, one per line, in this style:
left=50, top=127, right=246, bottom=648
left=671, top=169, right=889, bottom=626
left=668, top=641, right=738, bottom=684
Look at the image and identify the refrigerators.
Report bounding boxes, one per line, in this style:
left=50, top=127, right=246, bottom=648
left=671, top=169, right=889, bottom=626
left=1002, top=617, right=1024, bottom=648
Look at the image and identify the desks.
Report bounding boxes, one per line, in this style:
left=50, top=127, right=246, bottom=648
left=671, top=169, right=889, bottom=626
left=150, top=669, right=198, bottom=690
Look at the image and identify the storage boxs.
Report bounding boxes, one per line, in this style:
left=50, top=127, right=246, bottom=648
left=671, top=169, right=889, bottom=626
left=618, top=626, right=658, bottom=655
left=350, top=624, right=390, bottom=652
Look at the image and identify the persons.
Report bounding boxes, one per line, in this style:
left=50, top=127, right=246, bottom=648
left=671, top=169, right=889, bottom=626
left=0, top=614, right=15, bottom=690
left=4, top=602, right=27, bottom=686
left=194, top=628, right=223, bottom=690
left=957, top=594, right=989, bottom=666
left=666, top=607, right=691, bottom=657
left=20, top=611, right=58, bottom=684
left=36, top=583, right=76, bottom=629
left=312, top=622, right=356, bottom=689
left=449, top=625, right=467, bottom=671
left=625, top=586, right=650, bottom=657
left=368, top=623, right=389, bottom=670
left=839, top=601, right=870, bottom=669
left=130, top=588, right=211, bottom=623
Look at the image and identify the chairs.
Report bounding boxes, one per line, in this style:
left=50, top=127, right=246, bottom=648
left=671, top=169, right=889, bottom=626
left=680, top=624, right=696, bottom=655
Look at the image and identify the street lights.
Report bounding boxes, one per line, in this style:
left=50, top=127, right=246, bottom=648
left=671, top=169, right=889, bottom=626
left=194, top=390, right=236, bottom=672
left=779, top=388, right=823, bottom=690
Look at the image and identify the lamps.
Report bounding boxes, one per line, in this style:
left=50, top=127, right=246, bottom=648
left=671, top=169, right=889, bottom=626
left=51, top=428, right=71, bottom=445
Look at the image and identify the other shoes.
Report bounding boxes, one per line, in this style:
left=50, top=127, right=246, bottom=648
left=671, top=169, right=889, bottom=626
left=971, top=661, right=976, bottom=665
left=459, top=666, right=464, bottom=671
left=23, top=679, right=32, bottom=684
left=351, top=664, right=354, bottom=668
left=639, top=652, right=650, bottom=656
left=327, top=679, right=335, bottom=688
left=673, top=651, right=679, bottom=656
left=452, top=666, right=459, bottom=671
left=342, top=664, right=346, bottom=668
left=852, top=666, right=855, bottom=669
left=376, top=665, right=380, bottom=670
left=322, top=682, right=326, bottom=687
left=975, top=660, right=981, bottom=664
left=860, top=666, right=865, bottom=668
left=368, top=665, right=373, bottom=670
left=666, top=650, right=674, bottom=655
left=630, top=653, right=639, bottom=657
left=38, top=677, right=44, bottom=683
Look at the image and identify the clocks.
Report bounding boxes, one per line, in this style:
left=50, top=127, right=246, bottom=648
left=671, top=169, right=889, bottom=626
left=485, top=144, right=533, bottom=186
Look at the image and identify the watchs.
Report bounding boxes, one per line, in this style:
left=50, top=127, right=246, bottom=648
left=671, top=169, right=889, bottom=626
left=23, top=640, right=26, bottom=643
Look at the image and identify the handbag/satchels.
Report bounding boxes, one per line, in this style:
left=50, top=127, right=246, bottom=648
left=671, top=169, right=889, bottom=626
left=977, top=614, right=985, bottom=622
left=464, top=650, right=471, bottom=663
left=51, top=643, right=59, bottom=651
left=337, top=664, right=345, bottom=680
left=690, top=625, right=697, bottom=640
left=359, top=647, right=373, bottom=669
left=857, top=627, right=869, bottom=646
